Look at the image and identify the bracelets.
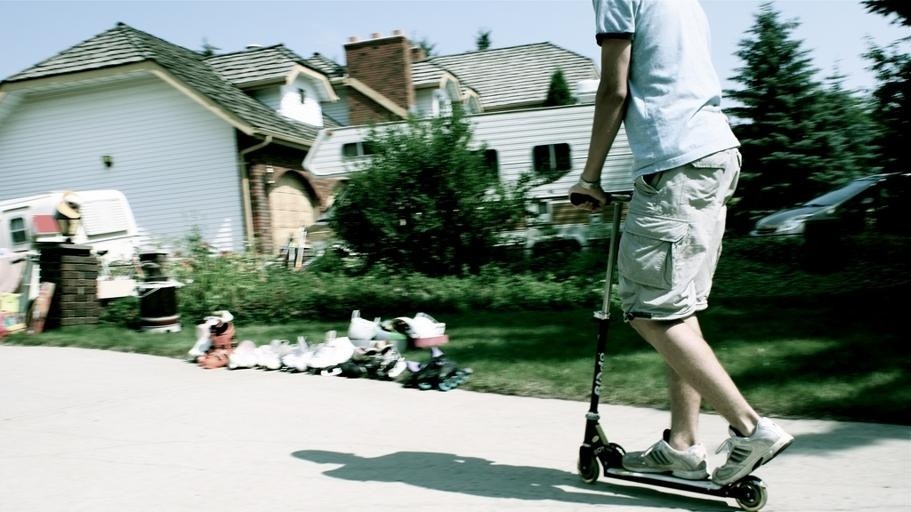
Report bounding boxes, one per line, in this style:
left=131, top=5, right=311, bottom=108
left=578, top=177, right=600, bottom=190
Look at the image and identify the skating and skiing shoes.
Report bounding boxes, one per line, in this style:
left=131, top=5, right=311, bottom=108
left=406, top=356, right=447, bottom=391
left=430, top=346, right=475, bottom=392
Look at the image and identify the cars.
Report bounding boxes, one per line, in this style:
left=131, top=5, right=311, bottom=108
left=746, top=169, right=910, bottom=242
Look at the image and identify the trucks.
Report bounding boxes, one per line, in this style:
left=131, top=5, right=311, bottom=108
left=302, top=102, right=633, bottom=279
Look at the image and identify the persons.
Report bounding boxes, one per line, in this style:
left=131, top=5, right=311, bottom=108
left=568, top=0, right=794, bottom=486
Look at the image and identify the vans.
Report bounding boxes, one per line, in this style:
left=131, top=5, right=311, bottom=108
left=0, top=184, right=146, bottom=307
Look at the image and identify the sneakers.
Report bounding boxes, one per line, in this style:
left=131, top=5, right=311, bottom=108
left=712, top=415, right=796, bottom=486
left=187, top=309, right=450, bottom=380
left=622, top=427, right=710, bottom=481
